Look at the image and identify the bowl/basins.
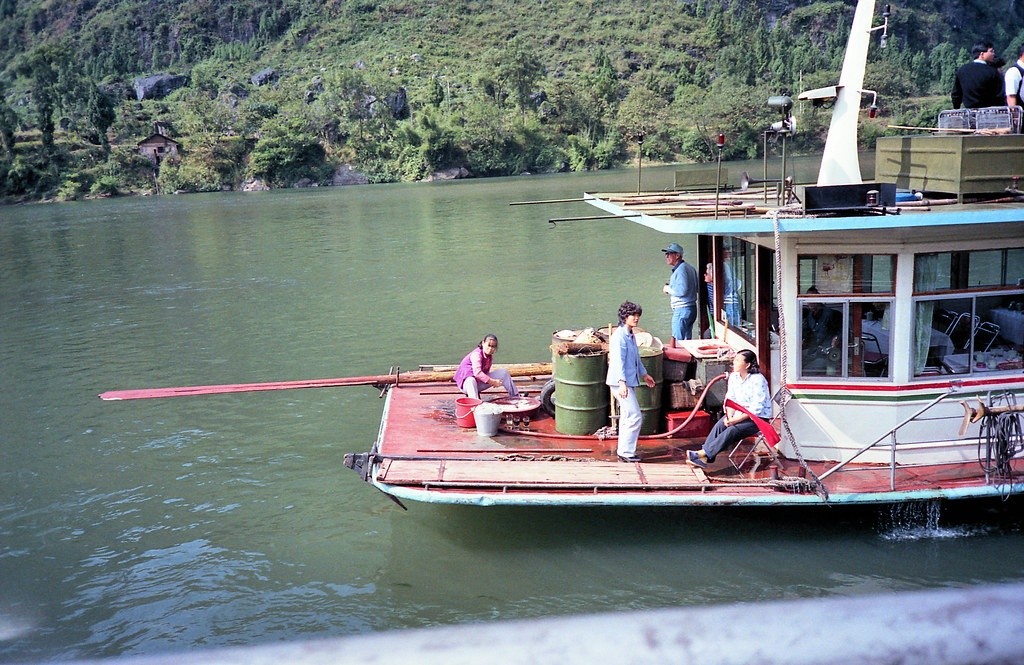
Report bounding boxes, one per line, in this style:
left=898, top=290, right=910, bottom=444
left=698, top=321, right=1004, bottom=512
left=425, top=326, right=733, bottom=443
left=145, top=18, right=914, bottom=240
left=489, top=397, right=542, bottom=422
left=991, top=349, right=1003, bottom=355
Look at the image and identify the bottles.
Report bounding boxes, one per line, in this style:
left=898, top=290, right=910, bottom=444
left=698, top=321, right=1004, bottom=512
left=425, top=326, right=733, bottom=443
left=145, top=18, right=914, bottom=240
left=867, top=312, right=872, bottom=320
left=1011, top=302, right=1023, bottom=310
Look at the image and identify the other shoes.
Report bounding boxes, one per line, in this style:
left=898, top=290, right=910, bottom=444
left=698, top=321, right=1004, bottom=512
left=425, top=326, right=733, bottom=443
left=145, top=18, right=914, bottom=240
left=686, top=450, right=699, bottom=460
left=686, top=458, right=707, bottom=469
left=618, top=454, right=641, bottom=462
left=518, top=392, right=529, bottom=397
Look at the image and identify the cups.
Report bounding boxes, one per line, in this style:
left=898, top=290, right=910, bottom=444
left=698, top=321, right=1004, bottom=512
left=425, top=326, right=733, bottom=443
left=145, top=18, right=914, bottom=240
left=523, top=416, right=529, bottom=427
left=977, top=351, right=985, bottom=362
left=1008, top=347, right=1018, bottom=359
left=513, top=415, right=520, bottom=426
left=1018, top=278, right=1024, bottom=288
left=506, top=414, right=513, bottom=426
left=987, top=355, right=996, bottom=369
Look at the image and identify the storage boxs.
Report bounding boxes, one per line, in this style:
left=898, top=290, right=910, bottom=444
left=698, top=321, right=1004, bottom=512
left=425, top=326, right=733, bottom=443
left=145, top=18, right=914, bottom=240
left=663, top=410, right=710, bottom=439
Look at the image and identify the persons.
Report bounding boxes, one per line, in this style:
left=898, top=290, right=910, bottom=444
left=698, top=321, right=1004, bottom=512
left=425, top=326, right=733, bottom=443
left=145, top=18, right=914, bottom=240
left=661, top=243, right=698, bottom=340
left=703, top=263, right=715, bottom=339
left=951, top=41, right=1003, bottom=134
left=1005, top=44, right=1024, bottom=134
left=801, top=285, right=842, bottom=377
left=722, top=239, right=742, bottom=325
left=453, top=334, right=521, bottom=400
left=606, top=301, right=655, bottom=462
left=685, top=350, right=773, bottom=469
left=987, top=55, right=1008, bottom=107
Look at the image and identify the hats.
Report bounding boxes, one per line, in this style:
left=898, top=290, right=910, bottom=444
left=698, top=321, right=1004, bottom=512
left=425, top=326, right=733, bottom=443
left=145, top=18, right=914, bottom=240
left=662, top=242, right=683, bottom=257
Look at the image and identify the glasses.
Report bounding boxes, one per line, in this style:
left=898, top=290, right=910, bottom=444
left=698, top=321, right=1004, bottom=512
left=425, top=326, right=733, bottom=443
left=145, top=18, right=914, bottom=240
left=705, top=271, right=711, bottom=276
left=665, top=252, right=678, bottom=258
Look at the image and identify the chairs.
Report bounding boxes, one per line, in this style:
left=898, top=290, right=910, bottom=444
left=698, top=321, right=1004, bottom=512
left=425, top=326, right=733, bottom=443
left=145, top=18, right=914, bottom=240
left=938, top=105, right=1023, bottom=133
left=729, top=387, right=794, bottom=479
left=851, top=330, right=888, bottom=378
left=922, top=307, right=1001, bottom=376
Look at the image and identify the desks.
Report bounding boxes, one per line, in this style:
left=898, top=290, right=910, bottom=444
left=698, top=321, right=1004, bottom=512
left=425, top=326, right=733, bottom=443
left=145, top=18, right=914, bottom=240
left=940, top=349, right=1023, bottom=374
left=862, top=319, right=955, bottom=364
left=989, top=307, right=1024, bottom=353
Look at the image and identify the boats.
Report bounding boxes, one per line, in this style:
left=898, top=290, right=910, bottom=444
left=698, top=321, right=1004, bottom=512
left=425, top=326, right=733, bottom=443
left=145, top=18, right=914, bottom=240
left=343, top=0, right=1024, bottom=508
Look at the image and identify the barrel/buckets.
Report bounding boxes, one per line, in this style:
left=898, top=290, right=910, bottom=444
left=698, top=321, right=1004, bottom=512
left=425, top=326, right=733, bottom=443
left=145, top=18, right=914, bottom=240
left=455, top=398, right=483, bottom=427
left=550, top=326, right=663, bottom=435
left=474, top=402, right=503, bottom=436
